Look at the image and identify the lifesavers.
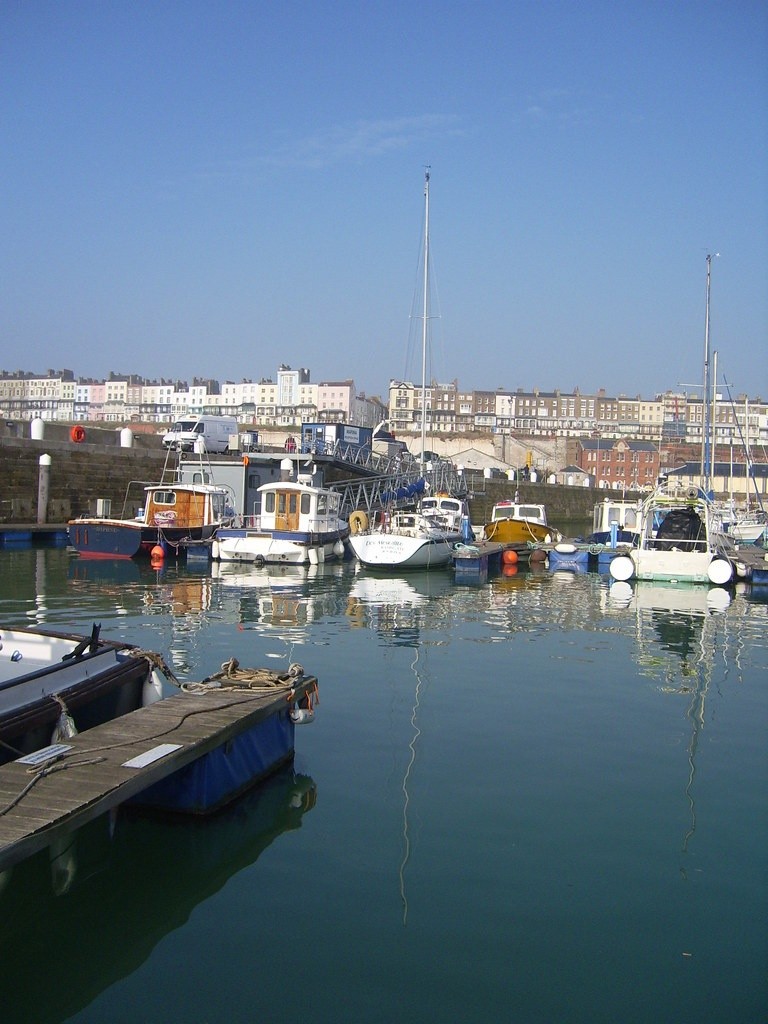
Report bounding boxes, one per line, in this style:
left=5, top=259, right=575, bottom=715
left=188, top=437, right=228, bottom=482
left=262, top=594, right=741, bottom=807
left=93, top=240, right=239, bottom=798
left=437, top=493, right=450, bottom=497
left=496, top=501, right=516, bottom=506
left=71, top=425, right=85, bottom=442
left=349, top=511, right=368, bottom=533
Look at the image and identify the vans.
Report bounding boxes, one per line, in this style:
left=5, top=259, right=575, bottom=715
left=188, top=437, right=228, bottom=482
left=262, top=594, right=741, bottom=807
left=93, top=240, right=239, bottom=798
left=162, top=414, right=238, bottom=455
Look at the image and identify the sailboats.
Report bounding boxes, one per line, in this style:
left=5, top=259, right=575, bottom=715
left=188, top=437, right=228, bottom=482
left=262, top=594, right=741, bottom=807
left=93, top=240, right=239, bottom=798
left=591, top=252, right=768, bottom=584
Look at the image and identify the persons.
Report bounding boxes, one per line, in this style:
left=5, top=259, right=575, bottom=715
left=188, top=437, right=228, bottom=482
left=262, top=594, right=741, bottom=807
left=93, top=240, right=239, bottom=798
left=521, top=464, right=535, bottom=481
left=285, top=433, right=297, bottom=453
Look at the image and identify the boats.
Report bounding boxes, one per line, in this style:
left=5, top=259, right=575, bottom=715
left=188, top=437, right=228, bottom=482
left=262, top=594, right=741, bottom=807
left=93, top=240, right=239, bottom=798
left=484, top=490, right=558, bottom=542
left=68, top=164, right=477, bottom=566
left=0, top=623, right=186, bottom=765
